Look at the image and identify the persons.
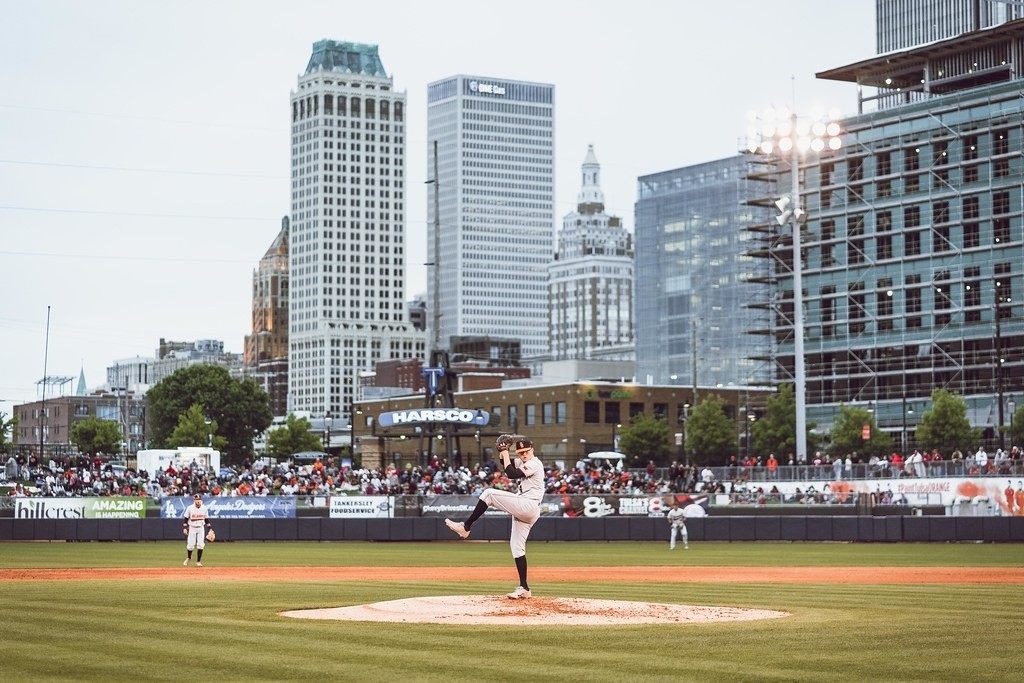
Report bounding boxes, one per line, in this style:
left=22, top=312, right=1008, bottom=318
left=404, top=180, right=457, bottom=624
left=904, top=445, right=1024, bottom=476
left=1005, top=480, right=1014, bottom=512
left=0, top=452, right=907, bottom=504
left=445, top=434, right=545, bottom=598
left=183, top=493, right=216, bottom=566
left=667, top=501, right=689, bottom=549
left=1013, top=481, right=1024, bottom=517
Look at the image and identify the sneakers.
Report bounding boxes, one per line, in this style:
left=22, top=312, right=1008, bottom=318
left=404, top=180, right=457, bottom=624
left=506, top=586, right=531, bottom=599
left=445, top=518, right=471, bottom=539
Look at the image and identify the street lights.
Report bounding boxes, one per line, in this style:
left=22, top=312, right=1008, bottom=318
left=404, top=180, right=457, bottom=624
left=612, top=420, right=623, bottom=452
left=324, top=411, right=335, bottom=467
left=346, top=402, right=364, bottom=469
left=1005, top=393, right=1017, bottom=449
left=204, top=416, right=213, bottom=446
left=901, top=396, right=915, bottom=461
left=866, top=401, right=875, bottom=455
left=745, top=404, right=756, bottom=456
left=677, top=402, right=692, bottom=467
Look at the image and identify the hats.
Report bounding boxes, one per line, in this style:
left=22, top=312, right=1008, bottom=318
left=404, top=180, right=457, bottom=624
left=194, top=494, right=202, bottom=500
left=673, top=501, right=679, bottom=505
left=516, top=440, right=533, bottom=454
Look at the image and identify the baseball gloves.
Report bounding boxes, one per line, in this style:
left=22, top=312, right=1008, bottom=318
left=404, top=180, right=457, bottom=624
left=206, top=529, right=216, bottom=542
left=495, top=433, right=513, bottom=452
left=678, top=523, right=683, bottom=529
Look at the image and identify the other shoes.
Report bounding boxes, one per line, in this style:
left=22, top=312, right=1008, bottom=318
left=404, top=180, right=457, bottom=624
left=195, top=562, right=203, bottom=567
left=685, top=546, right=688, bottom=550
left=183, top=558, right=190, bottom=566
left=670, top=547, right=674, bottom=550
